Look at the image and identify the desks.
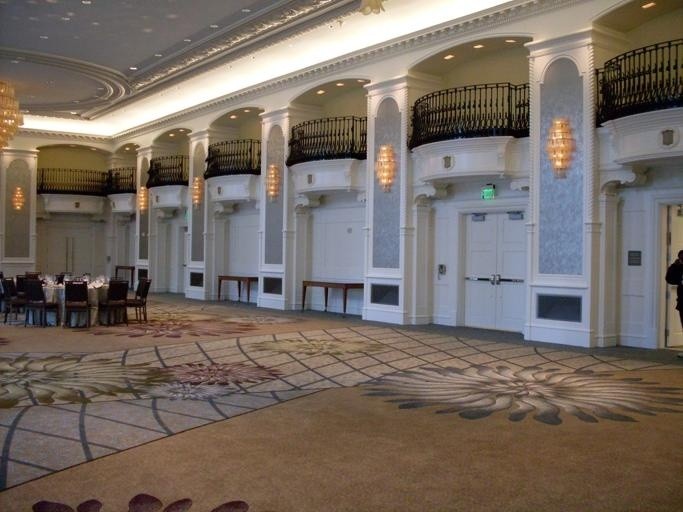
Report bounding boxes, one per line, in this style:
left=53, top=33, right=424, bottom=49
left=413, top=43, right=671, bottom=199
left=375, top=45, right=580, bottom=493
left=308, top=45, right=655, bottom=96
left=299, top=278, right=366, bottom=315
left=217, top=274, right=258, bottom=303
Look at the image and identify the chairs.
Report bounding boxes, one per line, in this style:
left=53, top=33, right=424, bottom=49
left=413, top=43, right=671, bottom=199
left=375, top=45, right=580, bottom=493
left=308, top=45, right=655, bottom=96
left=0, top=269, right=152, bottom=325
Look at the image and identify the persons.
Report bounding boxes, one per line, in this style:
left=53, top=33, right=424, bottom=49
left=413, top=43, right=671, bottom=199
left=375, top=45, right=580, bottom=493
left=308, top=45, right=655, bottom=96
left=665, top=250, right=683, bottom=356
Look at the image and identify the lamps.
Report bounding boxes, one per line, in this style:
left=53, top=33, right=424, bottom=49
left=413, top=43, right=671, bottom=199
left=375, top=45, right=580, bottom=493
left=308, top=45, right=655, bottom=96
left=265, top=164, right=278, bottom=200
left=14, top=186, right=26, bottom=212
left=192, top=177, right=202, bottom=210
left=0, top=79, right=24, bottom=153
left=138, top=184, right=149, bottom=213
left=549, top=115, right=572, bottom=179
left=376, top=143, right=396, bottom=193
